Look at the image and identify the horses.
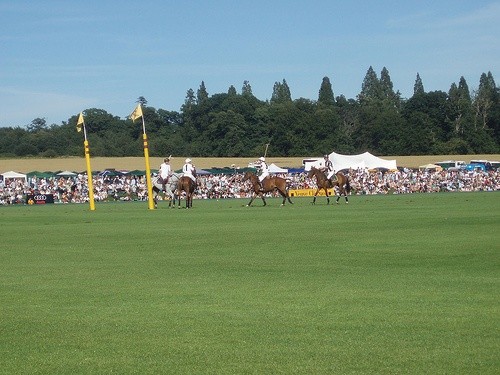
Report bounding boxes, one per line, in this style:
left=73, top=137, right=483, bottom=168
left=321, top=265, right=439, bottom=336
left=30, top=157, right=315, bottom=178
left=150, top=174, right=182, bottom=209
left=240, top=171, right=295, bottom=207
left=176, top=168, right=199, bottom=209
left=307, top=166, right=352, bottom=206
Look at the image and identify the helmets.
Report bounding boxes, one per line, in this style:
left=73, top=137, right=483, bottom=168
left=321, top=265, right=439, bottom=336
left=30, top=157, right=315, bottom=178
left=164, top=158, right=168, bottom=161
left=324, top=154, right=329, bottom=158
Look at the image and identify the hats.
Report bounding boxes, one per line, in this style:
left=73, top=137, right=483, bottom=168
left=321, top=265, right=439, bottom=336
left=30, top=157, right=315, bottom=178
left=258, top=156, right=265, bottom=161
left=185, top=158, right=191, bottom=163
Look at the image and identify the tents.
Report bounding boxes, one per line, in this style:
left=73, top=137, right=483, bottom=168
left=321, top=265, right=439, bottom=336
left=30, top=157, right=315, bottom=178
left=1, top=171, right=27, bottom=184
left=305, top=151, right=397, bottom=171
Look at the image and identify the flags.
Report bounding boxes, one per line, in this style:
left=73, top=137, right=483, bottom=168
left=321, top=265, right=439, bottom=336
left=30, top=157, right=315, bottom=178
left=129, top=104, right=142, bottom=122
left=76, top=112, right=85, bottom=133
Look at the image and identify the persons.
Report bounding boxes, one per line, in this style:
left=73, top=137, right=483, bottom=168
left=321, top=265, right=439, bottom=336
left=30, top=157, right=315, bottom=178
left=0, top=162, right=500, bottom=205
left=157, top=158, right=170, bottom=194
left=320, top=154, right=334, bottom=188
left=257, top=157, right=269, bottom=192
left=181, top=158, right=198, bottom=194
